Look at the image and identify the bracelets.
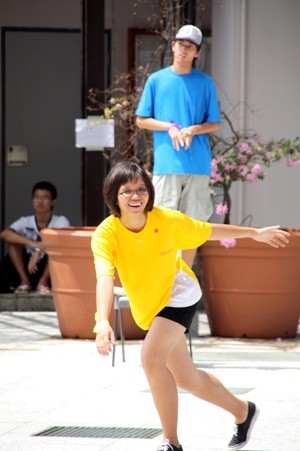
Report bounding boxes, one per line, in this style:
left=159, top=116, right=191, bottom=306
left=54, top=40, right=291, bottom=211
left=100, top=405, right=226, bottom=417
left=167, top=124, right=181, bottom=131
left=93, top=320, right=110, bottom=334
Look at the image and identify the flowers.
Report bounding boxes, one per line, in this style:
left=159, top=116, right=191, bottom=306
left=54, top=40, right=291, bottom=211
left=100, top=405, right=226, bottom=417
left=208, top=129, right=300, bottom=249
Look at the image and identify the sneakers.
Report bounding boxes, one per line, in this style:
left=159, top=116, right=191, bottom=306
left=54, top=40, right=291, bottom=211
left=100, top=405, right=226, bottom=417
left=228, top=401, right=260, bottom=451
left=156, top=439, right=183, bottom=451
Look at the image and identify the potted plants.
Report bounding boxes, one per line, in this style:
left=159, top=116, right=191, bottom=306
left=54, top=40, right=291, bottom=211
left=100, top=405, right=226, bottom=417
left=37, top=0, right=213, bottom=341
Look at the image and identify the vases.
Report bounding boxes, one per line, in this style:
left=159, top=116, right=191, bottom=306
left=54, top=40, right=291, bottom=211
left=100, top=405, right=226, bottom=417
left=196, top=229, right=300, bottom=341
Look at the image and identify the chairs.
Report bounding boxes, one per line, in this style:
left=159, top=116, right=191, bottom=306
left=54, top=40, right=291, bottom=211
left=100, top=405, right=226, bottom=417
left=111, top=292, right=191, bottom=369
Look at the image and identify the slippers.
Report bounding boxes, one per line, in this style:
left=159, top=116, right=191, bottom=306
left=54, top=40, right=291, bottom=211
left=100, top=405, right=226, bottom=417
left=36, top=284, right=50, bottom=293
left=13, top=285, right=32, bottom=293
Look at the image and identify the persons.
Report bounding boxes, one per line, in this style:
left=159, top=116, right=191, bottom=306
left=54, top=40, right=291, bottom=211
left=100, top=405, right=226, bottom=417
left=135, top=25, right=222, bottom=268
left=0, top=182, right=73, bottom=294
left=91, top=160, right=291, bottom=451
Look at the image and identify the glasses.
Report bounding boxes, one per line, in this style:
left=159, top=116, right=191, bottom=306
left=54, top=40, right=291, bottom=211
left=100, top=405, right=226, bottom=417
left=119, top=187, right=149, bottom=197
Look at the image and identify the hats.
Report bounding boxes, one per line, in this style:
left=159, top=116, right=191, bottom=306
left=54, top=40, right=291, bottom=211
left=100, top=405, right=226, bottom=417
left=175, top=25, right=204, bottom=49
